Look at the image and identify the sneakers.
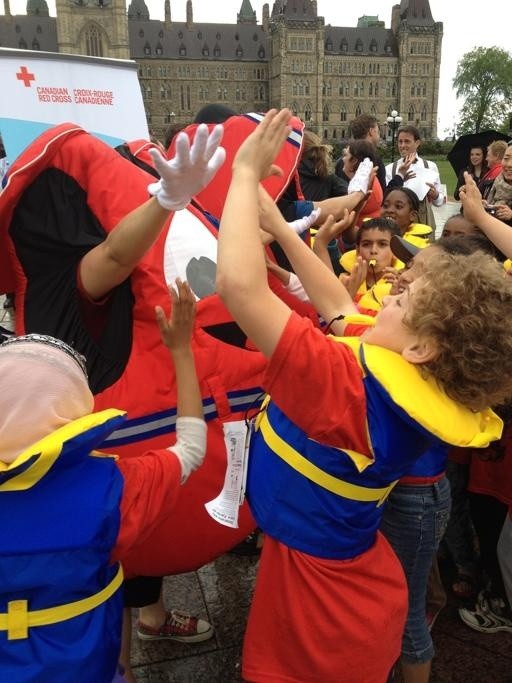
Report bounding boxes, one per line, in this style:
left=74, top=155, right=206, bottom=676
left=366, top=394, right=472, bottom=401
left=136, top=611, right=214, bottom=644
left=423, top=579, right=511, bottom=635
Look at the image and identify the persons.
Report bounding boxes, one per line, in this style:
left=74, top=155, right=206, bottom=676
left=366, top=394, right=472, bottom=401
left=2, top=101, right=512, bottom=682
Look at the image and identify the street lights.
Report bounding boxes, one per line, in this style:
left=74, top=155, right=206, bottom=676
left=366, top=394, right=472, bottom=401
left=384, top=108, right=403, bottom=163
left=169, top=110, right=176, bottom=123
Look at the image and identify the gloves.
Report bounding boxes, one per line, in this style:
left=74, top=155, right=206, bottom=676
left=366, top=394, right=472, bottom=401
left=288, top=208, right=321, bottom=236
left=147, top=122, right=226, bottom=211
left=347, top=158, right=373, bottom=198
